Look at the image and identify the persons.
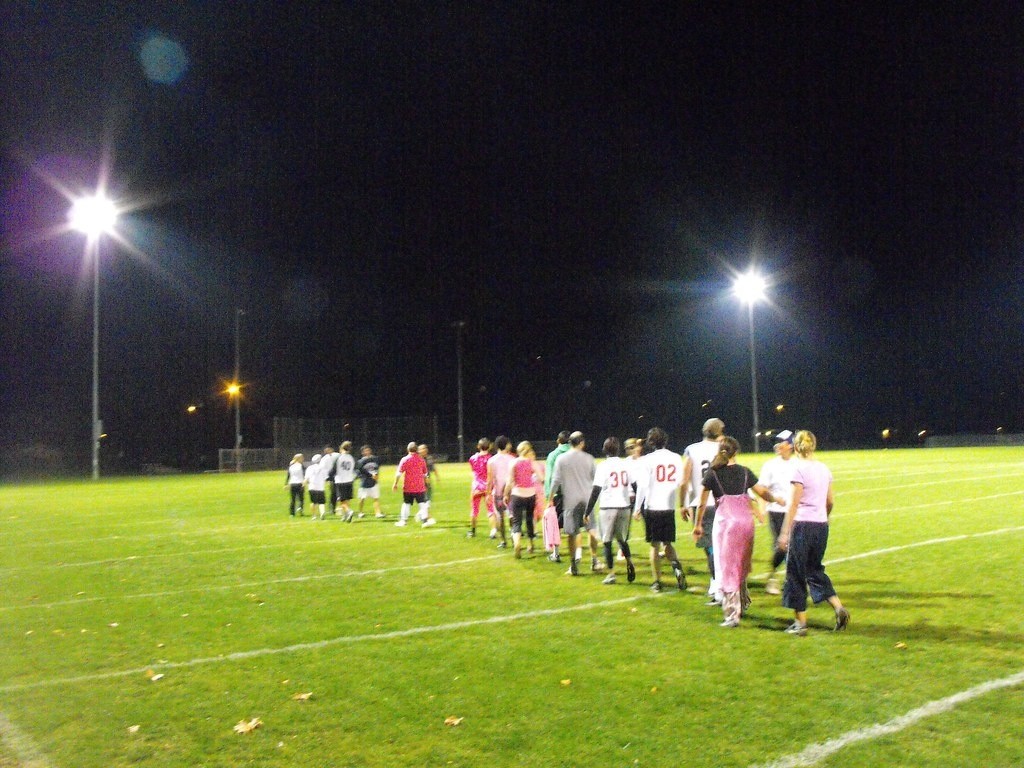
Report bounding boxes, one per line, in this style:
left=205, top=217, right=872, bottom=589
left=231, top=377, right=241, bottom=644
left=392, top=442, right=441, bottom=528
left=757, top=430, right=801, bottom=595
left=777, top=430, right=851, bottom=638
left=680, top=417, right=758, bottom=607
left=283, top=440, right=387, bottom=523
left=691, top=436, right=787, bottom=628
left=467, top=427, right=688, bottom=593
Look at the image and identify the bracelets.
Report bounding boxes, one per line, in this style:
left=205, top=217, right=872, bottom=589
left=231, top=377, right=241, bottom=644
left=394, top=481, right=397, bottom=484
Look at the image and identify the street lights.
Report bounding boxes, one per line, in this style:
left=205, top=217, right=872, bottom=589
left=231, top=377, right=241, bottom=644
left=450, top=320, right=467, bottom=462
left=730, top=271, right=772, bottom=451
left=65, top=195, right=119, bottom=482
left=235, top=309, right=249, bottom=473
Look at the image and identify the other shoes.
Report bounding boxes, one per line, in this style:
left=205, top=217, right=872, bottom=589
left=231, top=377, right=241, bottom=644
left=358, top=512, right=366, bottom=518
left=565, top=567, right=578, bottom=575
left=784, top=622, right=807, bottom=635
left=626, top=562, right=636, bottom=583
left=833, top=609, right=850, bottom=633
left=526, top=546, right=533, bottom=553
left=420, top=519, right=435, bottom=528
left=375, top=511, right=386, bottom=519
left=591, top=561, right=605, bottom=571
left=675, top=567, right=688, bottom=591
left=514, top=544, right=521, bottom=559
left=649, top=580, right=663, bottom=591
left=547, top=554, right=561, bottom=562
left=765, top=578, right=780, bottom=595
left=496, top=542, right=506, bottom=548
left=601, top=574, right=616, bottom=584
left=341, top=509, right=354, bottom=522
left=704, top=598, right=720, bottom=606
left=467, top=530, right=475, bottom=537
left=395, top=520, right=406, bottom=526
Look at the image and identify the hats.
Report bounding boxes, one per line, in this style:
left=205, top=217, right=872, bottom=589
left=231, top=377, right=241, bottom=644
left=777, top=430, right=797, bottom=446
left=623, top=437, right=643, bottom=450
left=311, top=454, right=321, bottom=463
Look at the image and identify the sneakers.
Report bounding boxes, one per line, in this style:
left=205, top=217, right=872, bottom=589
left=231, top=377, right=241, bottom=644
left=297, top=507, right=304, bottom=516
